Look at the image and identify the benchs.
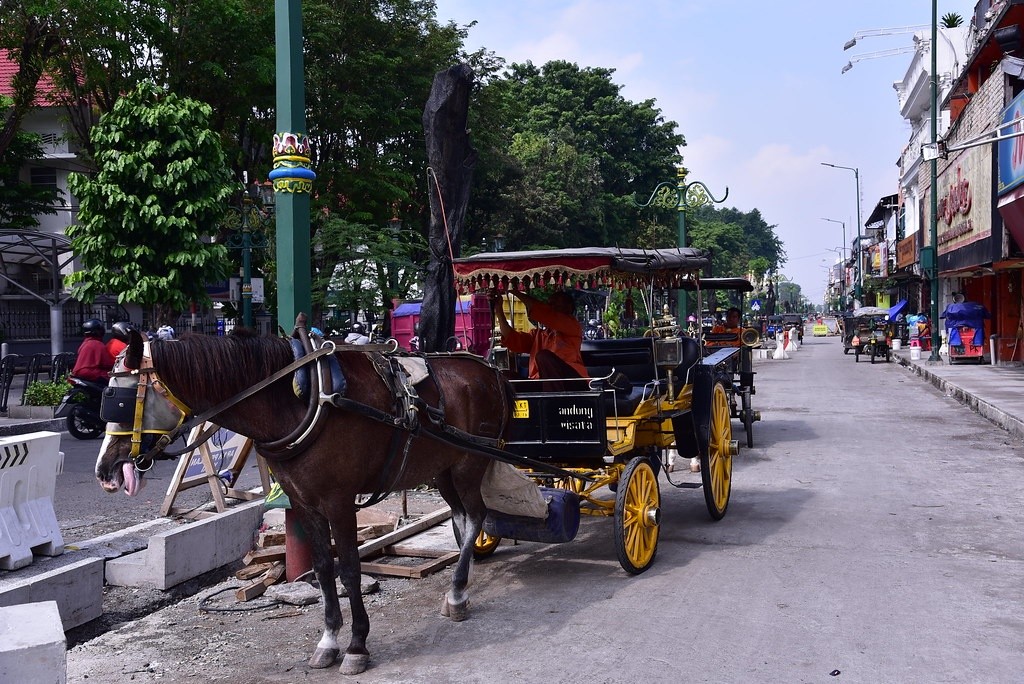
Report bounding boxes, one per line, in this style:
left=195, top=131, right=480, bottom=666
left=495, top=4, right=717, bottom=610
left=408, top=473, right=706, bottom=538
left=516, top=338, right=740, bottom=417
left=703, top=333, right=739, bottom=354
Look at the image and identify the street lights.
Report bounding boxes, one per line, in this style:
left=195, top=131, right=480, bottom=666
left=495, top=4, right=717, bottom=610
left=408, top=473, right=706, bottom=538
left=820, top=162, right=862, bottom=307
left=821, top=218, right=847, bottom=313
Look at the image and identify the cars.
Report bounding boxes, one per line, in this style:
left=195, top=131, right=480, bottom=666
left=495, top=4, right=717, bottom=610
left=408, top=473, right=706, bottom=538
left=742, top=312, right=802, bottom=338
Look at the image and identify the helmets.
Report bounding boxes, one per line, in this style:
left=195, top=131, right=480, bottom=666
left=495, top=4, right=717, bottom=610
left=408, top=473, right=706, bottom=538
left=589, top=319, right=599, bottom=326
left=81, top=318, right=105, bottom=337
left=111, top=322, right=133, bottom=342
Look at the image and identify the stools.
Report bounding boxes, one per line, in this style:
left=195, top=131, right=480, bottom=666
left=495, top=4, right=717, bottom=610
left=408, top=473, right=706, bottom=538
left=911, top=338, right=931, bottom=351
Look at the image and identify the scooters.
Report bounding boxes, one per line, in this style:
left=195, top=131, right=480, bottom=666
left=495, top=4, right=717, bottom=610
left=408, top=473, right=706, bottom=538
left=53, top=374, right=109, bottom=441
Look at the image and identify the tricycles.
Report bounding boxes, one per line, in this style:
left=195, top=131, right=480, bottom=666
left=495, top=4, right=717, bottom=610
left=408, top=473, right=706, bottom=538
left=854, top=320, right=894, bottom=366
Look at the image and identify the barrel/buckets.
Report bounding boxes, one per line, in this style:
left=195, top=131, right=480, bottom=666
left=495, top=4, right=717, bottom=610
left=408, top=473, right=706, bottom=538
left=910, top=347, right=921, bottom=360
left=892, top=339, right=901, bottom=350
left=481, top=487, right=580, bottom=543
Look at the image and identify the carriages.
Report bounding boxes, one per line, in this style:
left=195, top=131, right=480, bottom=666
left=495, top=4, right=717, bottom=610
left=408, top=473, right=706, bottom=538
left=94, top=240, right=763, bottom=675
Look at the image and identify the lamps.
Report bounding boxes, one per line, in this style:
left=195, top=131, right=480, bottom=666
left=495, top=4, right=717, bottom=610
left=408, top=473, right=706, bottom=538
left=984, top=0, right=1003, bottom=22
left=842, top=45, right=931, bottom=75
left=843, top=24, right=932, bottom=50
left=993, top=24, right=1021, bottom=55
left=920, top=116, right=1024, bottom=162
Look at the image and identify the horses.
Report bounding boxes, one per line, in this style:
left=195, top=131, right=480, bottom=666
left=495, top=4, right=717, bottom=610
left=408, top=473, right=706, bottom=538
left=95, top=331, right=520, bottom=676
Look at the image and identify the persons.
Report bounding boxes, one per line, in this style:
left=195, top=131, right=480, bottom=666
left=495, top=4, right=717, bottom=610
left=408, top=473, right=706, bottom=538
left=147, top=319, right=175, bottom=341
left=323, top=327, right=332, bottom=340
left=818, top=317, right=822, bottom=324
left=495, top=289, right=590, bottom=392
left=345, top=323, right=369, bottom=345
left=598, top=329, right=603, bottom=339
left=767, top=280, right=774, bottom=298
left=707, top=307, right=746, bottom=347
left=75, top=318, right=130, bottom=384
